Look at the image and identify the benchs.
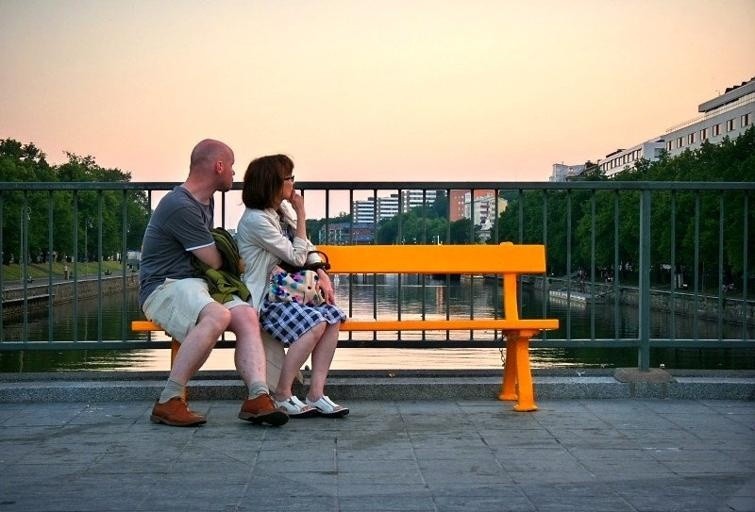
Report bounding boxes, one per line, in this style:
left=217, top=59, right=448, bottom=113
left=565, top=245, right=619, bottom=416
left=131, top=241, right=557, bottom=412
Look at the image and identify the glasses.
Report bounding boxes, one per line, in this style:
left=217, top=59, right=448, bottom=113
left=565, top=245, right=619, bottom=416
left=283, top=174, right=296, bottom=183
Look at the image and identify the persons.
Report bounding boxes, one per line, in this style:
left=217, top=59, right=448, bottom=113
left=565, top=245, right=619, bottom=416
left=236, top=154, right=352, bottom=417
left=136, top=140, right=290, bottom=427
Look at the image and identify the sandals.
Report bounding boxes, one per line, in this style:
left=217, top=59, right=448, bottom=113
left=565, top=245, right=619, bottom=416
left=272, top=395, right=316, bottom=418
left=304, top=395, right=350, bottom=416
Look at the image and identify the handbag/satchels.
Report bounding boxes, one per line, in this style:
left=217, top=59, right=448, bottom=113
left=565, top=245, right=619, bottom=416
left=261, top=249, right=332, bottom=306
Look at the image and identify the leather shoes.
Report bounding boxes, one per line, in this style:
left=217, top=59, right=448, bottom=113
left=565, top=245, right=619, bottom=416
left=150, top=395, right=207, bottom=426
left=237, top=392, right=290, bottom=427
